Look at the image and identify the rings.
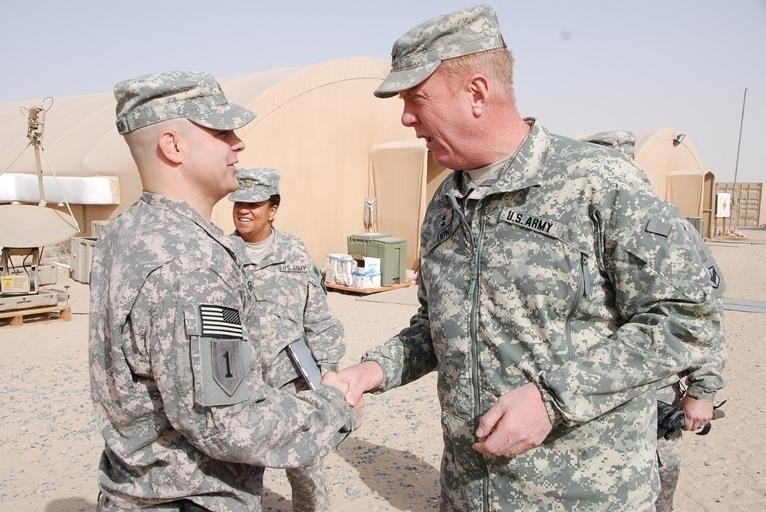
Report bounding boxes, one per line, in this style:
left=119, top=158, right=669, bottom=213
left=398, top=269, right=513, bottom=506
left=699, top=424, right=705, bottom=428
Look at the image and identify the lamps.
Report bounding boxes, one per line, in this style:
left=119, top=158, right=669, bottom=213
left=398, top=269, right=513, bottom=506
left=674, top=134, right=686, bottom=144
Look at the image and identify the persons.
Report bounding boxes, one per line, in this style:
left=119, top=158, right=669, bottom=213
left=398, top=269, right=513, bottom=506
left=581, top=130, right=726, bottom=512
left=330, top=4, right=717, bottom=512
left=85, top=71, right=365, bottom=512
left=223, top=167, right=349, bottom=512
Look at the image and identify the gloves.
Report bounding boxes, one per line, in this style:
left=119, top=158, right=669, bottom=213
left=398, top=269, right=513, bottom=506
left=657, top=399, right=725, bottom=440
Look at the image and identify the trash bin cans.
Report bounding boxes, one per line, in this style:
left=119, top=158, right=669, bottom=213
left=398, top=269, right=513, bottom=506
left=347, top=233, right=407, bottom=287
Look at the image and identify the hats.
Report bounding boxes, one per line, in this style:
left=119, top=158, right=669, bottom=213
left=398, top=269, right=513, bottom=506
left=229, top=167, right=281, bottom=204
left=373, top=4, right=507, bottom=99
left=113, top=68, right=257, bottom=135
